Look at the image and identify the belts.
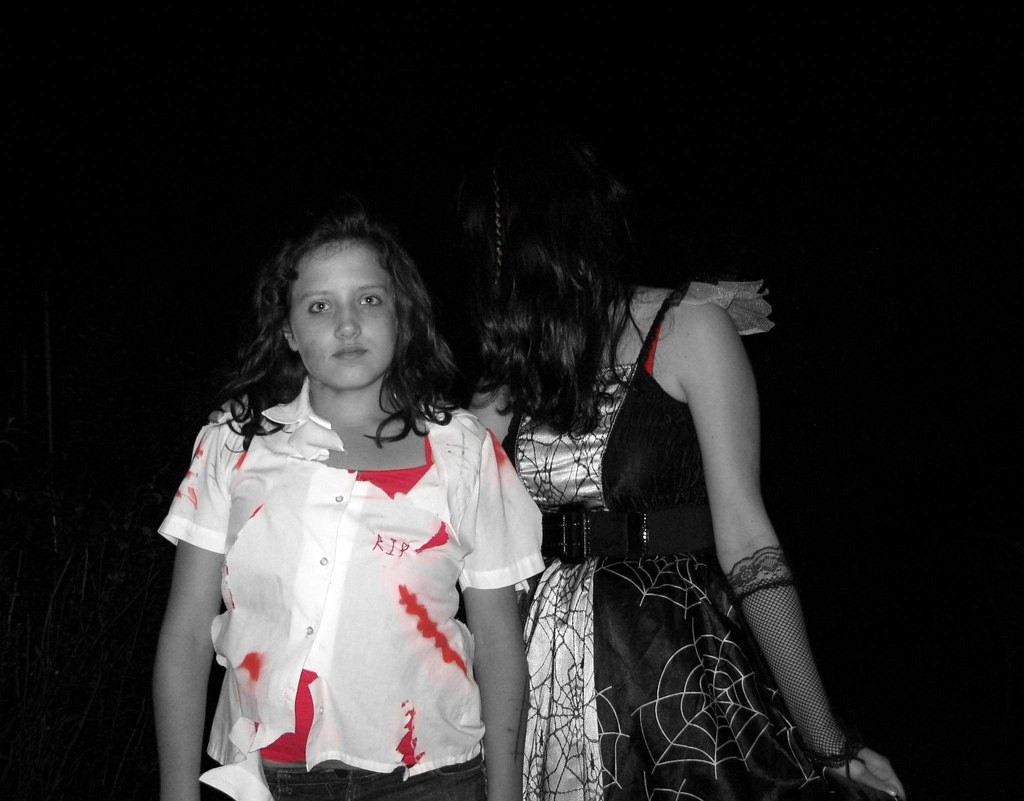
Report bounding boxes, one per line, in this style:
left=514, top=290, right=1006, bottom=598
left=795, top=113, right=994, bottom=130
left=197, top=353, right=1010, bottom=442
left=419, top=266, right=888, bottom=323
left=541, top=501, right=713, bottom=565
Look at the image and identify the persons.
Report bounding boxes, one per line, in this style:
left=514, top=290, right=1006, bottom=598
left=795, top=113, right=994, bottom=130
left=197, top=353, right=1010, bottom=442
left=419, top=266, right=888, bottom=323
left=143, top=211, right=542, bottom=800
left=457, top=115, right=911, bottom=800
